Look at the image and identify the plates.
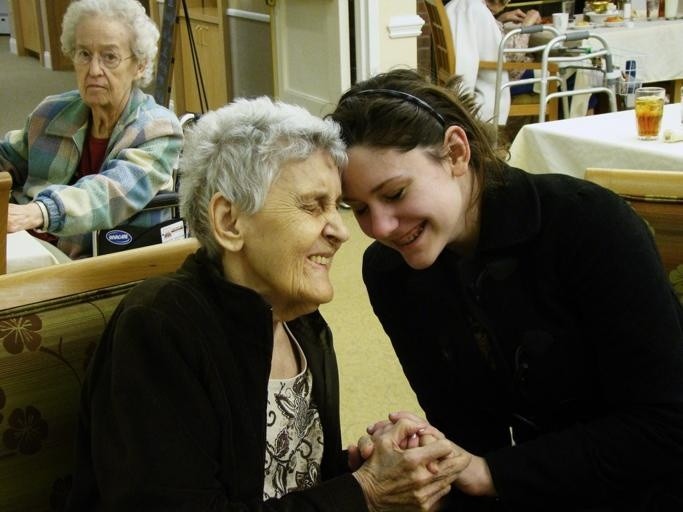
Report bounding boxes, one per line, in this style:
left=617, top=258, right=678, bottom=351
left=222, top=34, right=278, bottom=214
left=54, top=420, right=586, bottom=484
left=574, top=1, right=622, bottom=31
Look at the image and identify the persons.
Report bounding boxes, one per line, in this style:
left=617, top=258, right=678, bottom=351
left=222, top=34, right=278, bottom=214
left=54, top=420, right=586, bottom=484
left=445, top=2, right=598, bottom=117
left=72, top=93, right=475, bottom=511
left=321, top=67, right=683, bottom=510
left=0, top=0, right=183, bottom=261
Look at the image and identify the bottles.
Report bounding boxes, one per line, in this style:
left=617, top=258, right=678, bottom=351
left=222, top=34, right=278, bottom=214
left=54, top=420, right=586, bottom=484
left=623, top=0, right=632, bottom=19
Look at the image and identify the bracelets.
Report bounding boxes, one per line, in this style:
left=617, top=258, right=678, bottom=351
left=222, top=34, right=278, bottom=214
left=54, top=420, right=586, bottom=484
left=33, top=197, right=48, bottom=235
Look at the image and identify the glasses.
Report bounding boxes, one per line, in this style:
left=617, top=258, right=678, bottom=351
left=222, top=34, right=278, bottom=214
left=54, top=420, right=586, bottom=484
left=70, top=42, right=136, bottom=71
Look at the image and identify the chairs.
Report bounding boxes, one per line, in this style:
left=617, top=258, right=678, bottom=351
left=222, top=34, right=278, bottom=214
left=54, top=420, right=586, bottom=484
left=2, top=237, right=199, bottom=509
left=584, top=167, right=683, bottom=309
left=423, top=0, right=558, bottom=121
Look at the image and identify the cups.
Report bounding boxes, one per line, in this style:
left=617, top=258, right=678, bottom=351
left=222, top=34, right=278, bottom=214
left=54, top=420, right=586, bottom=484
left=551, top=13, right=569, bottom=35
left=562, top=1, right=575, bottom=23
left=634, top=88, right=666, bottom=140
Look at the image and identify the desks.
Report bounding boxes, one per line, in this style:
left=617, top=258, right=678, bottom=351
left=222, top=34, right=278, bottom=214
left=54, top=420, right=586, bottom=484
left=524, top=12, right=683, bottom=121
left=503, top=102, right=683, bottom=180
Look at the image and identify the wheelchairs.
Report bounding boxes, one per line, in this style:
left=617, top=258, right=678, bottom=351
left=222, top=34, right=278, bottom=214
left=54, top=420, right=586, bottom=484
left=86, top=110, right=204, bottom=257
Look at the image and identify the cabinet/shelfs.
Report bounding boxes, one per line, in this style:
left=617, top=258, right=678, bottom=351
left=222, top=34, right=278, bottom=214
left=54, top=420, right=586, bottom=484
left=178, top=11, right=225, bottom=119
